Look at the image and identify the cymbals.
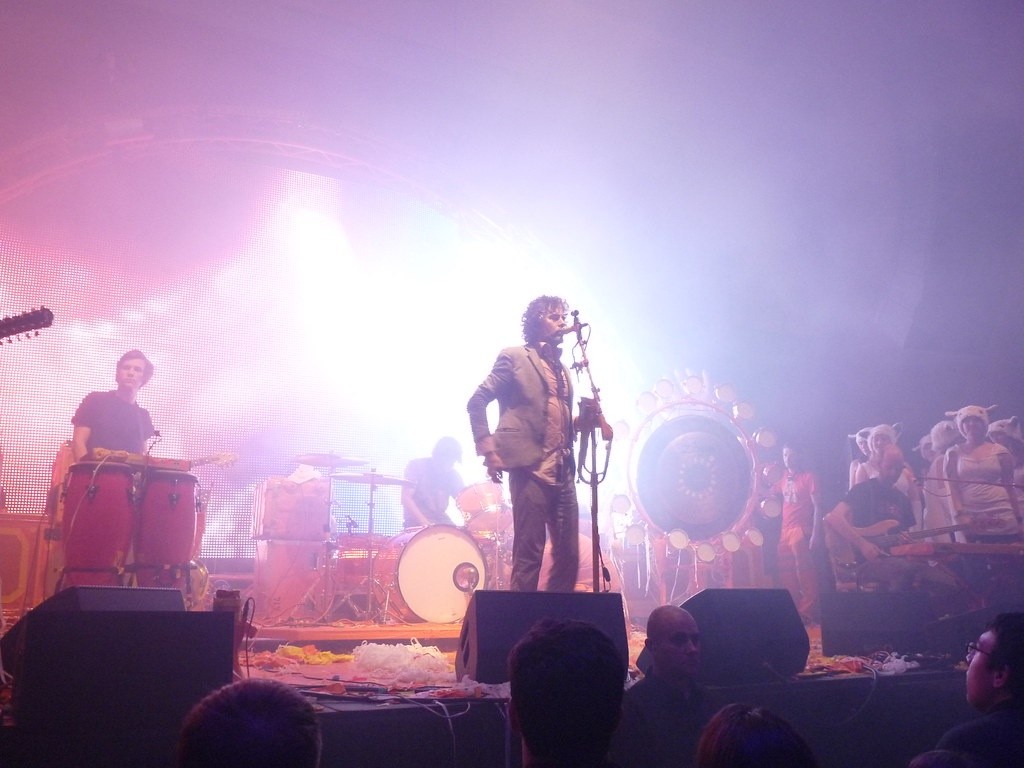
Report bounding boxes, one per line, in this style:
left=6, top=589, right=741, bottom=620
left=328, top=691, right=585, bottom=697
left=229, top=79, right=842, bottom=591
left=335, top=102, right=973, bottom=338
left=328, top=472, right=416, bottom=487
left=293, top=454, right=370, bottom=467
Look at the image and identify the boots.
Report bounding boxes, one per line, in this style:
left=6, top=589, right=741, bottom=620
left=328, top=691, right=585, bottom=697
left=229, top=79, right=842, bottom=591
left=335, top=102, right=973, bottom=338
left=805, top=625, right=823, bottom=671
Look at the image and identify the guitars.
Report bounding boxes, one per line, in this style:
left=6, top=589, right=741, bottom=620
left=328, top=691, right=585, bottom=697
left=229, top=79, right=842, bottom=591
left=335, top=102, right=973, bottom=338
left=827, top=515, right=1011, bottom=590
left=190, top=450, right=241, bottom=472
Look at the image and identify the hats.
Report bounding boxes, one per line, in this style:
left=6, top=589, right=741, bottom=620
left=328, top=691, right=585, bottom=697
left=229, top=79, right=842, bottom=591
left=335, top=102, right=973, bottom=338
left=848, top=422, right=904, bottom=451
left=913, top=405, right=1024, bottom=458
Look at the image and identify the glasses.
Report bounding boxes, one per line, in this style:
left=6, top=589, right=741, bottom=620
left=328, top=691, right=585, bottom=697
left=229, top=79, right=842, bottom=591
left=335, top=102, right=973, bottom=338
left=968, top=641, right=993, bottom=660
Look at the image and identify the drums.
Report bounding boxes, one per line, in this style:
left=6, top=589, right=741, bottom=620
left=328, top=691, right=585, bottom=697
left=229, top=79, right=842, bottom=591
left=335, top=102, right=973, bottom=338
left=63, top=459, right=199, bottom=604
left=453, top=477, right=513, bottom=534
left=372, top=519, right=490, bottom=623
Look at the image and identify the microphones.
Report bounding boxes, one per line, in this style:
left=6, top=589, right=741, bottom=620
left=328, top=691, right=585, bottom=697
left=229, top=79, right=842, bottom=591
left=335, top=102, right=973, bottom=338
left=901, top=466, right=922, bottom=486
left=561, top=322, right=588, bottom=334
left=240, top=600, right=250, bottom=643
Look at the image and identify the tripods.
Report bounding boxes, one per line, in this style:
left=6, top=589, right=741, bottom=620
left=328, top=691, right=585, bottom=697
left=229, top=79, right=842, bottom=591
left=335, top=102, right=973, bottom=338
left=287, top=464, right=408, bottom=625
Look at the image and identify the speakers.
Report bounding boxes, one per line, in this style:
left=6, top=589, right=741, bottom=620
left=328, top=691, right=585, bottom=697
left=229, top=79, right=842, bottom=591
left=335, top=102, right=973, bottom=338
left=637, top=587, right=811, bottom=682
left=1, top=586, right=240, bottom=728
left=818, top=589, right=948, bottom=658
left=454, top=588, right=629, bottom=685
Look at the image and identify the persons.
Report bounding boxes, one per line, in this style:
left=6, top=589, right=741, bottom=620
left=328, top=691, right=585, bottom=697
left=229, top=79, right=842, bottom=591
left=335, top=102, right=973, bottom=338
left=401, top=437, right=468, bottom=531
left=907, top=611, right=1024, bottom=768
left=507, top=605, right=825, bottom=768
left=72, top=350, right=155, bottom=463
left=761, top=404, right=1024, bottom=625
left=173, top=679, right=323, bottom=768
left=467, top=295, right=582, bottom=592
